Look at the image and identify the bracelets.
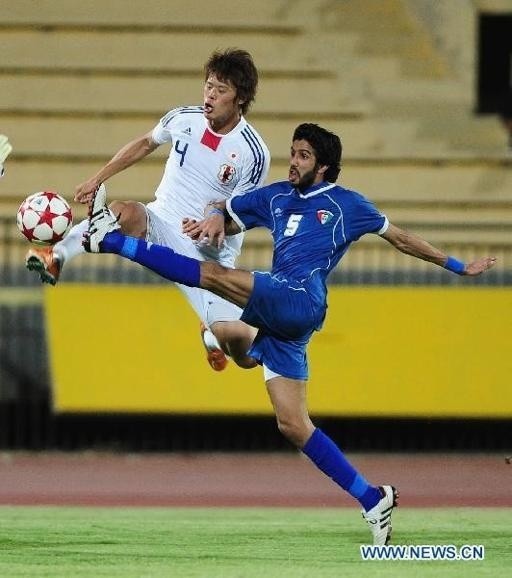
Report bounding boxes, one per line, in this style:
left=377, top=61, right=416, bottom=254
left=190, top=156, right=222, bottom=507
left=442, top=254, right=469, bottom=278
left=206, top=209, right=226, bottom=218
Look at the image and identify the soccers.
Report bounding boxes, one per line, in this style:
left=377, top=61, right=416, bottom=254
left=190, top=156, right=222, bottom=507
left=17, top=192, right=72, bottom=245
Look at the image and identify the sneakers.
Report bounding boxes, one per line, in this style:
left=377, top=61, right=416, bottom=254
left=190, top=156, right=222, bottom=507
left=81, top=181, right=122, bottom=255
left=200, top=322, right=228, bottom=372
left=360, top=484, right=398, bottom=555
left=24, top=246, right=62, bottom=287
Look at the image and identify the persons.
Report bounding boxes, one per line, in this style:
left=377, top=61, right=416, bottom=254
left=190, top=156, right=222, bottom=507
left=81, top=124, right=498, bottom=548
left=23, top=48, right=269, bottom=371
